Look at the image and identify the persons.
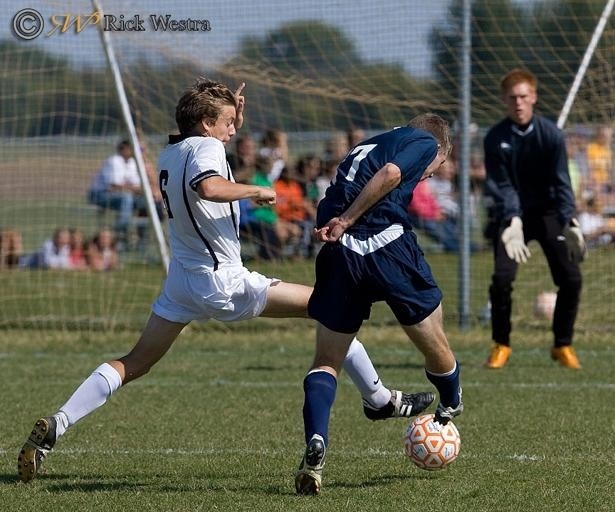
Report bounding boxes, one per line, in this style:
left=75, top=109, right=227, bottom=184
left=292, top=112, right=466, bottom=494
left=0, top=125, right=615, bottom=277
left=480, top=68, right=587, bottom=372
left=15, top=76, right=437, bottom=485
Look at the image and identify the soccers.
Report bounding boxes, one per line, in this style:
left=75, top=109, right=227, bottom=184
left=535, top=292, right=557, bottom=320
left=404, top=414, right=460, bottom=470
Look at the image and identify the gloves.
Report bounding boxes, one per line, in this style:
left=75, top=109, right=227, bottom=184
left=556, top=222, right=589, bottom=264
left=501, top=216, right=532, bottom=264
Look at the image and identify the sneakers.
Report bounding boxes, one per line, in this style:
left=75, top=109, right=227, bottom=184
left=485, top=343, right=512, bottom=369
left=551, top=345, right=582, bottom=369
left=434, top=386, right=463, bottom=426
left=18, top=417, right=57, bottom=483
left=295, top=434, right=326, bottom=495
left=362, top=389, right=434, bottom=422
